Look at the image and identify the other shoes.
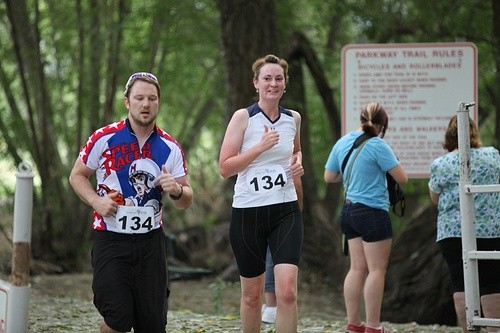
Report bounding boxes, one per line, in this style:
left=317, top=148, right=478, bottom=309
left=345, top=322, right=365, bottom=333
left=364, top=325, right=394, bottom=333
left=261, top=304, right=277, bottom=323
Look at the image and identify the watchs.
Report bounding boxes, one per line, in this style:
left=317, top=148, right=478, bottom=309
left=169, top=185, right=183, bottom=200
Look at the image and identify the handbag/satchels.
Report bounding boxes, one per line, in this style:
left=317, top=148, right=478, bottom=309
left=338, top=231, right=347, bottom=255
left=386, top=158, right=405, bottom=217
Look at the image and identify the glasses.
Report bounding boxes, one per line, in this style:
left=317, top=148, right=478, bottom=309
left=130, top=73, right=158, bottom=80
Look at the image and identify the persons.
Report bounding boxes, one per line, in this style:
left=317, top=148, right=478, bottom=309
left=218, top=54, right=305, bottom=333
left=69, top=72, right=194, bottom=333
left=323, top=102, right=409, bottom=333
left=427, top=115, right=500, bottom=333
